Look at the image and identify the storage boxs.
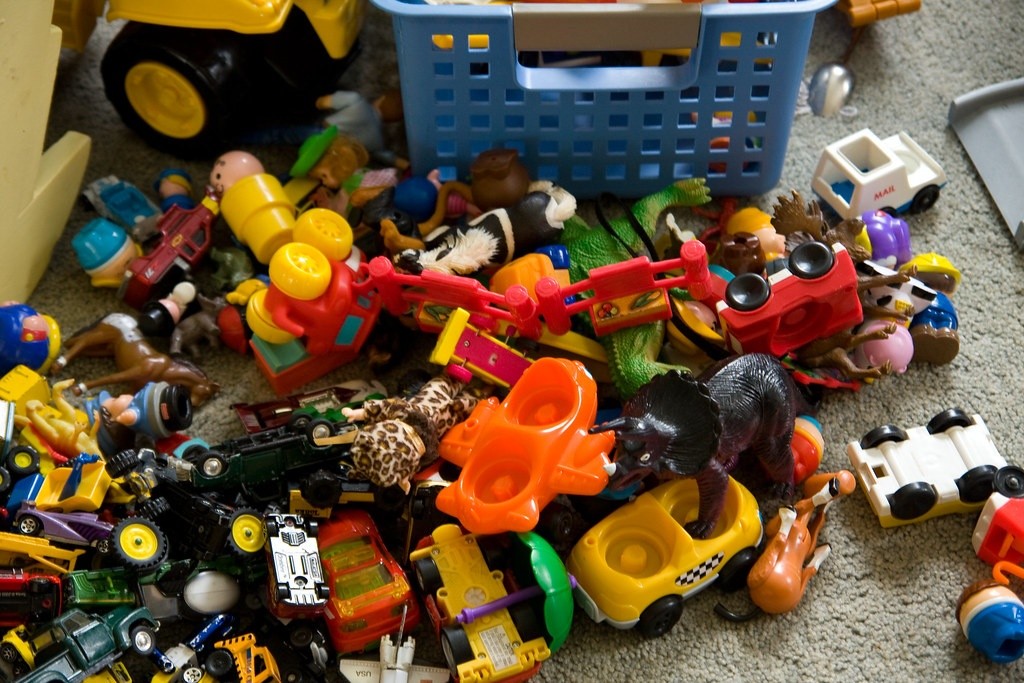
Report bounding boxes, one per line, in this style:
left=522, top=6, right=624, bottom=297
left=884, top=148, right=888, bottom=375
left=370, top=0, right=839, bottom=202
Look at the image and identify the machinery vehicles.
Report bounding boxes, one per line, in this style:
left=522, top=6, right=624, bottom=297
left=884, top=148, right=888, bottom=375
left=99, top=0, right=775, bottom=163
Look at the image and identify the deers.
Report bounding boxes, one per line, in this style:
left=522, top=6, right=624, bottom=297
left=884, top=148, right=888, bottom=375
left=769, top=191, right=918, bottom=380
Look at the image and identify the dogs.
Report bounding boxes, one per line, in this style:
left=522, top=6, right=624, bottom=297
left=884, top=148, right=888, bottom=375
left=392, top=180, right=577, bottom=275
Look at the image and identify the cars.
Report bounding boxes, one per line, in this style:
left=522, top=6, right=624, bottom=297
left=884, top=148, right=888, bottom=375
left=565, top=476, right=764, bottom=639
left=971, top=466, right=1024, bottom=586
left=715, top=240, right=863, bottom=354
left=0, top=405, right=558, bottom=683
left=116, top=195, right=219, bottom=303
left=847, top=409, right=1013, bottom=528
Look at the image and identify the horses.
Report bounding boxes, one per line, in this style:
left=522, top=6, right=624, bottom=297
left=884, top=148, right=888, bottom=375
left=51, top=312, right=222, bottom=406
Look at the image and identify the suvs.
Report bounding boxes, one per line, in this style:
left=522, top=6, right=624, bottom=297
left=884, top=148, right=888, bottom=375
left=810, top=128, right=948, bottom=220
